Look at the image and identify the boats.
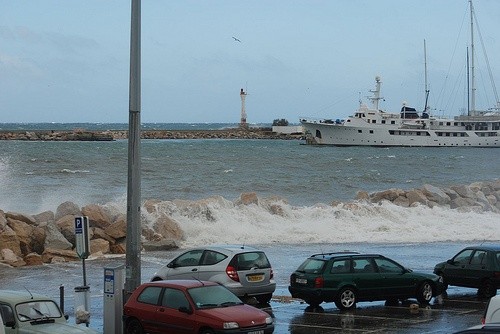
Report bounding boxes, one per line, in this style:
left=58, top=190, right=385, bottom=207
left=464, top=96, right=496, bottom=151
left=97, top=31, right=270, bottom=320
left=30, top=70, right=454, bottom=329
left=300, top=74, right=500, bottom=148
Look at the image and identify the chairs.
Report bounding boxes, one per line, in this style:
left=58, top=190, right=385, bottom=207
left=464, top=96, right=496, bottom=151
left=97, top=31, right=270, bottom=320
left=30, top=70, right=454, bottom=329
left=364, top=264, right=373, bottom=273
left=478, top=253, right=485, bottom=259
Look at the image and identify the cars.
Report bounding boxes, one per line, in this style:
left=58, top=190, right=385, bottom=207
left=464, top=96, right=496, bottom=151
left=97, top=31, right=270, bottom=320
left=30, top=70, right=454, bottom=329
left=433, top=242, right=500, bottom=289
left=149, top=244, right=276, bottom=304
left=124, top=279, right=276, bottom=334
left=0, top=288, right=97, bottom=334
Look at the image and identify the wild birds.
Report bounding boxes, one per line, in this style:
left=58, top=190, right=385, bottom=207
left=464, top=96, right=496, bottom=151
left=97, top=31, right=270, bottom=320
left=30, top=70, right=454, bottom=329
left=232, top=36, right=240, bottom=41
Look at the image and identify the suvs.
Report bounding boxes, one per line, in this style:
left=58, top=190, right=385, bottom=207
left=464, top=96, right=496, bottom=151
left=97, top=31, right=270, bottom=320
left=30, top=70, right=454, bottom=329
left=287, top=249, right=449, bottom=310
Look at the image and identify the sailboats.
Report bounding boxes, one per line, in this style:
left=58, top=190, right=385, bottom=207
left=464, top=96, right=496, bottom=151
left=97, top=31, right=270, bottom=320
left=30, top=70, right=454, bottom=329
left=428, top=0, right=500, bottom=121
